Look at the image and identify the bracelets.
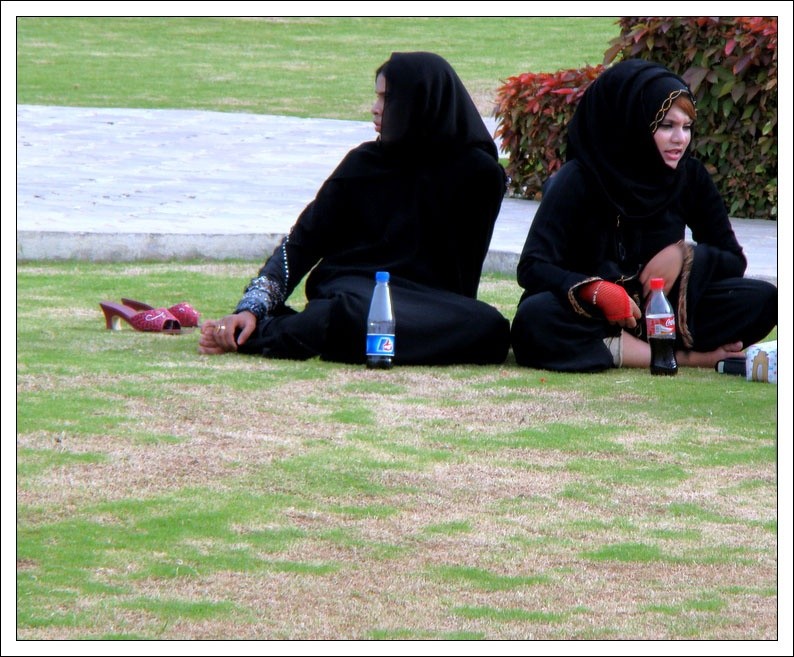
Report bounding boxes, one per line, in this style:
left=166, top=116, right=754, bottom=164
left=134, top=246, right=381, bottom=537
left=593, top=280, right=603, bottom=305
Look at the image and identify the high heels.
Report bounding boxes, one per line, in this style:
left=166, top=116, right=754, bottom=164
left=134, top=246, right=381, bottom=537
left=99, top=301, right=184, bottom=335
left=122, top=298, right=203, bottom=327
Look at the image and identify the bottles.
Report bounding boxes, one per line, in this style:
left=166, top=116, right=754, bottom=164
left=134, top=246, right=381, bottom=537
left=366, top=272, right=396, bottom=371
left=645, top=278, right=678, bottom=377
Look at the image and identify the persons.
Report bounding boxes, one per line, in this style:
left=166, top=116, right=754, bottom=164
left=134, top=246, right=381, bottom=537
left=198, top=51, right=511, bottom=368
left=511, top=58, right=778, bottom=373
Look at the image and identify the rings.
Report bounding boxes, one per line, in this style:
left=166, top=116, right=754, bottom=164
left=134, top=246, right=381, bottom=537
left=214, top=324, right=225, bottom=333
